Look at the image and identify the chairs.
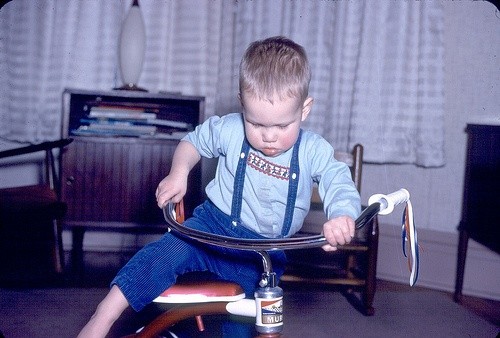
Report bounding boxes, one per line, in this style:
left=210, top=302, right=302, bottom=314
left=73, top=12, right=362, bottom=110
left=0, top=137, right=74, bottom=281
left=278, top=144, right=379, bottom=316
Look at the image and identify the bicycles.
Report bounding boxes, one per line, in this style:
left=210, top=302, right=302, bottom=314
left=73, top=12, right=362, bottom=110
left=110, top=186, right=422, bottom=338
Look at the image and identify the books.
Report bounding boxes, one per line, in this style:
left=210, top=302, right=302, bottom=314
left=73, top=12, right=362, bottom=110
left=70, top=97, right=194, bottom=141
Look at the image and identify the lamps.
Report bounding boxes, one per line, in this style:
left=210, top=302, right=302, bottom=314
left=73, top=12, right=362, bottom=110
left=112, top=0, right=148, bottom=92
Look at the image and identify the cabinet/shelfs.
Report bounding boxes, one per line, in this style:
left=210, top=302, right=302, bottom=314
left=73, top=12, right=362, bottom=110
left=59, top=89, right=205, bottom=268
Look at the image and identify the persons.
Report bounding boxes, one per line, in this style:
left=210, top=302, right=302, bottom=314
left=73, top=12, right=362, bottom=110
left=74, top=35, right=363, bottom=338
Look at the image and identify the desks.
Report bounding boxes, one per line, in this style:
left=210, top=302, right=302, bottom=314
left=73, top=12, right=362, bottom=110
left=454, top=124, right=500, bottom=304
